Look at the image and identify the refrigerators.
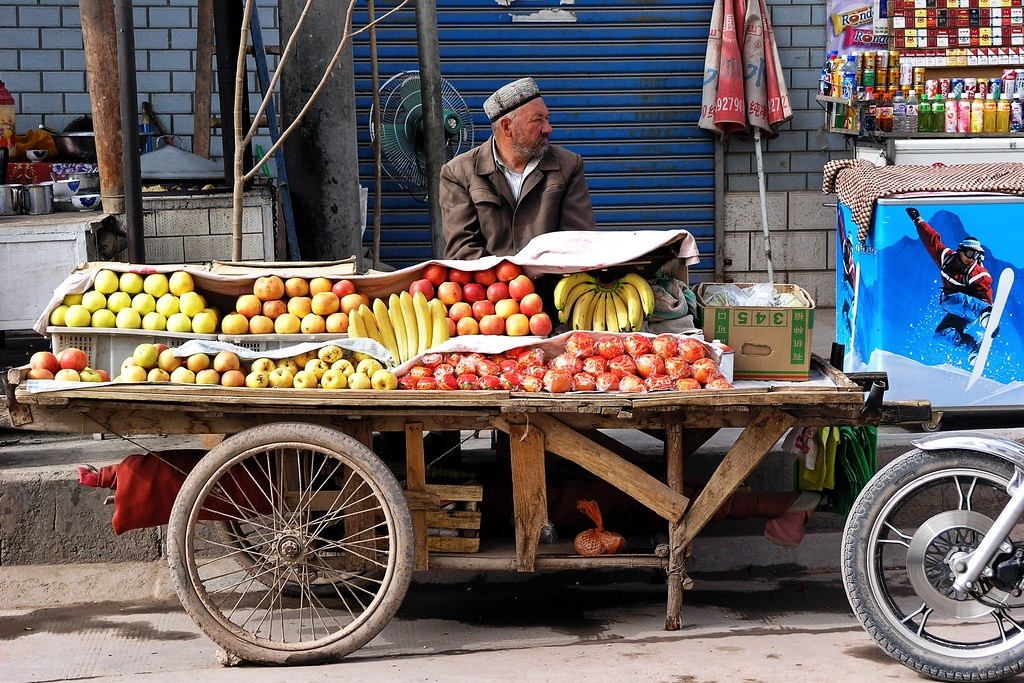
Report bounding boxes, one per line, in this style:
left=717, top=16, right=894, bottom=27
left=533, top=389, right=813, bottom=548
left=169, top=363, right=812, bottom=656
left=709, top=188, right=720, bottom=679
left=833, top=182, right=1023, bottom=406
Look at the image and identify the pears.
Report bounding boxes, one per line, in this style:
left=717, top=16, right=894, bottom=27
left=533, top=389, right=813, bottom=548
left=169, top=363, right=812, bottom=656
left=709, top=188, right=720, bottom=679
left=245, top=345, right=397, bottom=390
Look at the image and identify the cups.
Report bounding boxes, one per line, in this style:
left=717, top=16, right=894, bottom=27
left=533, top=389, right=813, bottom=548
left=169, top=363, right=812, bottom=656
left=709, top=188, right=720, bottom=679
left=18, top=184, right=55, bottom=215
left=0, top=184, right=24, bottom=217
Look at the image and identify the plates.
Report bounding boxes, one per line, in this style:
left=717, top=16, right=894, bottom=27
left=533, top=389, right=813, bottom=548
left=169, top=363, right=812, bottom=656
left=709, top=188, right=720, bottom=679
left=141, top=189, right=226, bottom=197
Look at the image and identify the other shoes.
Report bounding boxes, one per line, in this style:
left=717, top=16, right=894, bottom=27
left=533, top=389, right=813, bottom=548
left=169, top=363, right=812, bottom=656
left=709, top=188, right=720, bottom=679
left=968, top=338, right=982, bottom=360
left=979, top=312, right=992, bottom=327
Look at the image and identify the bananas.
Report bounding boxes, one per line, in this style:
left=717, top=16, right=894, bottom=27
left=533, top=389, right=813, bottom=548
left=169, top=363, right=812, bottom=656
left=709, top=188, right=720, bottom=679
left=347, top=291, right=449, bottom=365
left=554, top=273, right=654, bottom=331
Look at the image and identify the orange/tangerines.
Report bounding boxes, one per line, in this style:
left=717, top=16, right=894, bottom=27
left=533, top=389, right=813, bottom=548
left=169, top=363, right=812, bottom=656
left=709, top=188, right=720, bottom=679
left=398, top=333, right=731, bottom=393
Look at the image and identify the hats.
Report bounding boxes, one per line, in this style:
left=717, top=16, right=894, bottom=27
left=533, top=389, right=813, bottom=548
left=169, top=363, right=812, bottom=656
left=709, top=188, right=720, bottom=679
left=959, top=237, right=985, bottom=253
left=483, top=78, right=540, bottom=122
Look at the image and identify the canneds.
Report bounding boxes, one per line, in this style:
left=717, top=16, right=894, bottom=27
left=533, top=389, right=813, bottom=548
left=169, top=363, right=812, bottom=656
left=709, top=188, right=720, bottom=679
left=925, top=77, right=1003, bottom=100
left=850, top=49, right=925, bottom=98
left=999, top=68, right=1024, bottom=99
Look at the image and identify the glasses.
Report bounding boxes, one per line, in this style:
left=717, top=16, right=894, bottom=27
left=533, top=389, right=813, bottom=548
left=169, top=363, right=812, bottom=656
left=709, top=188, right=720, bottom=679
left=965, top=250, right=982, bottom=259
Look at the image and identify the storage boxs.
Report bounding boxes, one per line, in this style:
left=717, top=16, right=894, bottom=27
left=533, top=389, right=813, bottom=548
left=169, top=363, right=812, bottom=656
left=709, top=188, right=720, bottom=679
left=44, top=326, right=349, bottom=383
left=692, top=281, right=816, bottom=382
left=712, top=449, right=802, bottom=515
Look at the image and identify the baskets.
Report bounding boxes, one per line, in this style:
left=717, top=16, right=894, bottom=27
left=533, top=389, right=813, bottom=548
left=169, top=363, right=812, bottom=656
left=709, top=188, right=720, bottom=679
left=219, top=333, right=348, bottom=352
left=47, top=326, right=218, bottom=382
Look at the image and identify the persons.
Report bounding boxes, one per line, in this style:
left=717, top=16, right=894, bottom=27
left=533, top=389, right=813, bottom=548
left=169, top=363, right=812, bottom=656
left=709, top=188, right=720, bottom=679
left=837, top=210, right=857, bottom=338
left=904, top=207, right=1000, bottom=366
left=438, top=77, right=596, bottom=261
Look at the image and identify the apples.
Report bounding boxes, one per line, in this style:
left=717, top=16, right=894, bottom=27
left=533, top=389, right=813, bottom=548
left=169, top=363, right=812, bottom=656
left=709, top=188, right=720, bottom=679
left=409, top=261, right=551, bottom=335
left=26, top=348, right=111, bottom=382
left=222, top=276, right=366, bottom=335
left=113, top=344, right=247, bottom=387
left=49, top=269, right=219, bottom=333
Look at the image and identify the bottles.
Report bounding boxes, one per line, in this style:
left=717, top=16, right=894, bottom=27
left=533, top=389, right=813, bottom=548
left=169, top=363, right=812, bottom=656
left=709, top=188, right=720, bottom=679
left=851, top=86, right=1024, bottom=132
left=818, top=50, right=852, bottom=128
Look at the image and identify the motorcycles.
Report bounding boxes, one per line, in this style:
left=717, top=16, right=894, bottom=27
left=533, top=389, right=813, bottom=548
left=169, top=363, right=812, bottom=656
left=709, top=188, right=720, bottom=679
left=838, top=429, right=1024, bottom=682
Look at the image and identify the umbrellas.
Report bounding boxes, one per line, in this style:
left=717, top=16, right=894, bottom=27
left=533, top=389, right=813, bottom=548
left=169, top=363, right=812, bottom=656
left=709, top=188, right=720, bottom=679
left=697, top=0, right=794, bottom=283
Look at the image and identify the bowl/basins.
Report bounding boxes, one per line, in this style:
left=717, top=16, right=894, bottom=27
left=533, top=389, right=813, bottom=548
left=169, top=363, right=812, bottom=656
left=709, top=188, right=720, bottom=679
left=26, top=150, right=49, bottom=163
left=71, top=194, right=100, bottom=212
left=50, top=164, right=102, bottom=190
left=39, top=180, right=81, bottom=203
left=50, top=131, right=149, bottom=163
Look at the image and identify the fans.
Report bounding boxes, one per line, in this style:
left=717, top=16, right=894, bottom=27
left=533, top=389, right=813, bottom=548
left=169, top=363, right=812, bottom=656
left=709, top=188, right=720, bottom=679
left=368, top=70, right=474, bottom=205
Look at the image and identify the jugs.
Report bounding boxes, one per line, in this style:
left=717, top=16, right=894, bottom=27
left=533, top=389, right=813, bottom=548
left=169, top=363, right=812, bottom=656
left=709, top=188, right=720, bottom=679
left=0, top=80, right=19, bottom=163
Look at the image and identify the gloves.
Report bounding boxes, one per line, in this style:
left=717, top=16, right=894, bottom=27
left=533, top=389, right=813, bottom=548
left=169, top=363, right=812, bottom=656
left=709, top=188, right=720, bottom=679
left=906, top=208, right=920, bottom=222
